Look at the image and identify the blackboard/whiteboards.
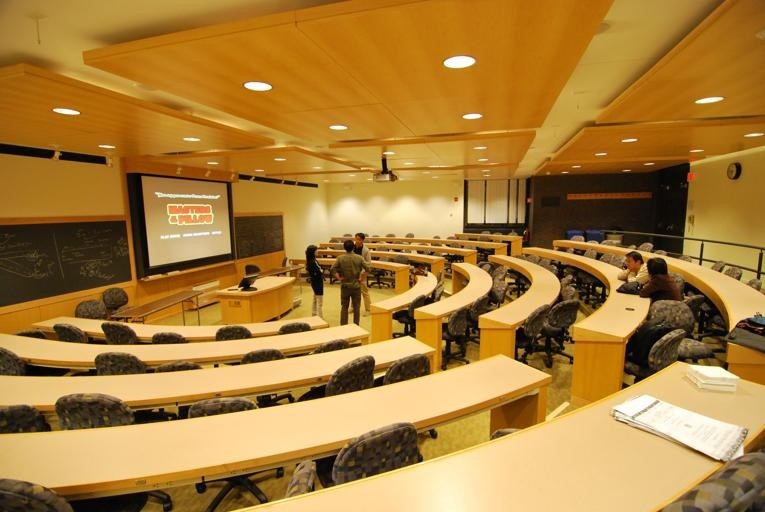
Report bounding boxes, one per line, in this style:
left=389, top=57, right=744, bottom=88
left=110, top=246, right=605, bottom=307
left=0, top=212, right=286, bottom=313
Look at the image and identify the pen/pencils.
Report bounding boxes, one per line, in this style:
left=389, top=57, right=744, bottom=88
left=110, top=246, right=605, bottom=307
left=228, top=289, right=238, bottom=291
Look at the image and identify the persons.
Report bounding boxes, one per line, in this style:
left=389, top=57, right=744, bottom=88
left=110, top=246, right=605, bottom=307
left=617, top=251, right=650, bottom=286
left=347, top=232, right=372, bottom=317
left=306, top=245, right=326, bottom=320
left=640, top=257, right=685, bottom=301
left=330, top=240, right=369, bottom=326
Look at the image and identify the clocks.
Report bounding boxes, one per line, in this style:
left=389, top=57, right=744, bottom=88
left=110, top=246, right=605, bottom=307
left=726, top=160, right=742, bottom=181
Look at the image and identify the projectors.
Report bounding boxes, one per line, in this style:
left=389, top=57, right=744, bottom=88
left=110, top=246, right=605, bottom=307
left=372, top=171, right=398, bottom=183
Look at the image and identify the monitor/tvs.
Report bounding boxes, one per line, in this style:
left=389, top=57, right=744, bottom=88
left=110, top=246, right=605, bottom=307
left=238, top=274, right=258, bottom=291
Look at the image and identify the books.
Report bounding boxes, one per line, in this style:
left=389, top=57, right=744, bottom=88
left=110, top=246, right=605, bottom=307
left=611, top=393, right=749, bottom=463
left=687, top=372, right=737, bottom=391
left=688, top=365, right=741, bottom=385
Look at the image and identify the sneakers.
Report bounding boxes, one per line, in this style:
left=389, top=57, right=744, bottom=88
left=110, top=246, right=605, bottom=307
left=347, top=307, right=372, bottom=317
